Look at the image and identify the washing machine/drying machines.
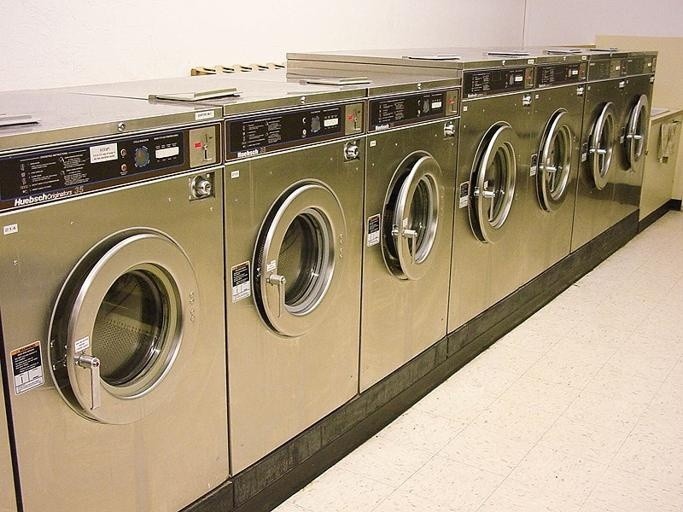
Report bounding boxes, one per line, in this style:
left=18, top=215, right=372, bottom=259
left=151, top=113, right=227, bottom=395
left=0, top=44, right=660, bottom=512
